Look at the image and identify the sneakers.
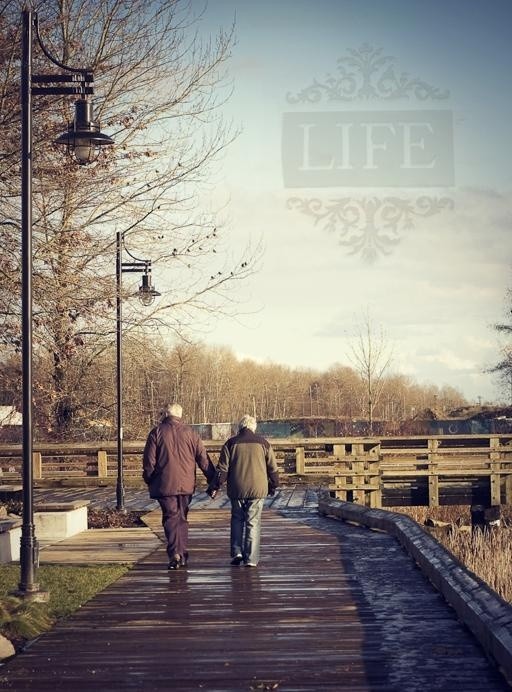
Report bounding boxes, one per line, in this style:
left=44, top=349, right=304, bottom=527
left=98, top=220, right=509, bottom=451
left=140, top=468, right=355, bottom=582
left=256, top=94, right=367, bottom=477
left=230, top=553, right=257, bottom=567
left=168, top=554, right=186, bottom=570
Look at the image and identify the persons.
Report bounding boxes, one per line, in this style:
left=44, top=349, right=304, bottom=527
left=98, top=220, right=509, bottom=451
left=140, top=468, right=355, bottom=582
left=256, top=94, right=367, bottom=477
left=207, top=414, right=278, bottom=567
left=142, top=403, right=220, bottom=570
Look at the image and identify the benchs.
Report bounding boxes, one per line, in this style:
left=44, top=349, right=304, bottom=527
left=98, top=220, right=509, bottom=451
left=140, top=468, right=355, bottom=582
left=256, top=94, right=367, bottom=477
left=0, top=514, right=29, bottom=567
left=5, top=497, right=96, bottom=545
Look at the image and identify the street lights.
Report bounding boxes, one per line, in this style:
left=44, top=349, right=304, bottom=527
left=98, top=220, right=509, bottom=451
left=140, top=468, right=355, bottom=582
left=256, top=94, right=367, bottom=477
left=110, top=232, right=162, bottom=514
left=13, top=11, right=114, bottom=610
